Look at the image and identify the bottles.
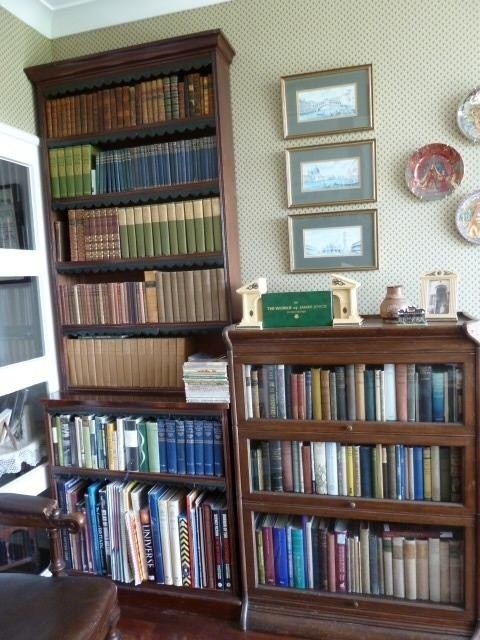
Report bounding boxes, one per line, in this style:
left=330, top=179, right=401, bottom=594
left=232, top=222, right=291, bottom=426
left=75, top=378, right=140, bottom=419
left=379, top=285, right=408, bottom=324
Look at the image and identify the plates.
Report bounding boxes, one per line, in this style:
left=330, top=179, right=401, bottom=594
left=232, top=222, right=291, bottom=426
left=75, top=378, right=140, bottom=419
left=454, top=192, right=480, bottom=245
left=404, top=143, right=464, bottom=200
left=456, top=85, right=480, bottom=143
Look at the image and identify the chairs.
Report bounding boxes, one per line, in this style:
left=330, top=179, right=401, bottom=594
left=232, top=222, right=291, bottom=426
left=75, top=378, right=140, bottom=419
left=1, top=491, right=123, bottom=639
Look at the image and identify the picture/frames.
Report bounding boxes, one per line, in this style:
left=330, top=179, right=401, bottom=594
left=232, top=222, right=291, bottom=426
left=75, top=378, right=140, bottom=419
left=279, top=64, right=379, bottom=273
left=420, top=270, right=458, bottom=322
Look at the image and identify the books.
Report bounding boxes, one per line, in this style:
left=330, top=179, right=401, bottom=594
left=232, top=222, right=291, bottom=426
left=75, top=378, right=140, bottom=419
left=0, top=283, right=41, bottom=366
left=250, top=440, right=462, bottom=504
left=242, top=362, right=464, bottom=423
left=254, top=512, right=465, bottom=605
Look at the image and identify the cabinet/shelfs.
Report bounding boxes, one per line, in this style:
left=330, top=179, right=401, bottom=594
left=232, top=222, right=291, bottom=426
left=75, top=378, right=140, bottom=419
left=1, top=122, right=62, bottom=577
left=23, top=29, right=240, bottom=623
left=224, top=312, right=480, bottom=635
